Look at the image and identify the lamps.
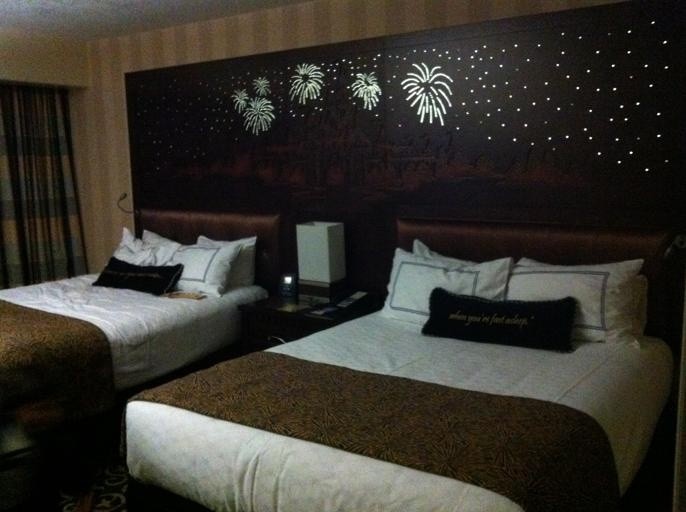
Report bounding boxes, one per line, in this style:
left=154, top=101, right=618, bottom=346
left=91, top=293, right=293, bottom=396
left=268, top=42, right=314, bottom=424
left=295, top=221, right=348, bottom=297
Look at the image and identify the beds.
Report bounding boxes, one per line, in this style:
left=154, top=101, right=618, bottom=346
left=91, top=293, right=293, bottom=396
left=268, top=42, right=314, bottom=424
left=0, top=209, right=280, bottom=461
left=119, top=214, right=686, bottom=512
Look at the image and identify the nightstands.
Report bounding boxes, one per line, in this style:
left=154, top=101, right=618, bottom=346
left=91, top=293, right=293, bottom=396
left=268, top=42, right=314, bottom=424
left=238, top=287, right=380, bottom=358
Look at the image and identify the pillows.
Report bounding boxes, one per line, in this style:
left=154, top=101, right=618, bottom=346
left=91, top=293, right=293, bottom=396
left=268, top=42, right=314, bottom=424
left=92, top=225, right=258, bottom=297
left=382, top=238, right=648, bottom=349
